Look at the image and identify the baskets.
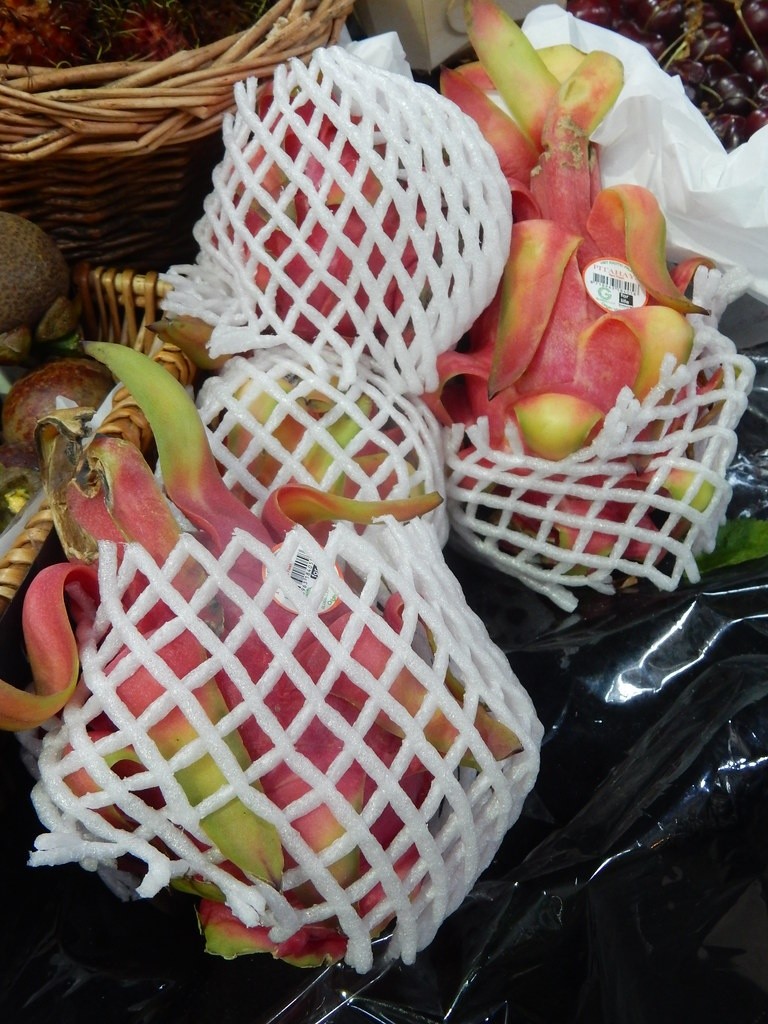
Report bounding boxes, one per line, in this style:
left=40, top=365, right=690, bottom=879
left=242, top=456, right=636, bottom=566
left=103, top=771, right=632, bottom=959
left=0, top=263, right=200, bottom=629
left=0, top=0, right=359, bottom=276
